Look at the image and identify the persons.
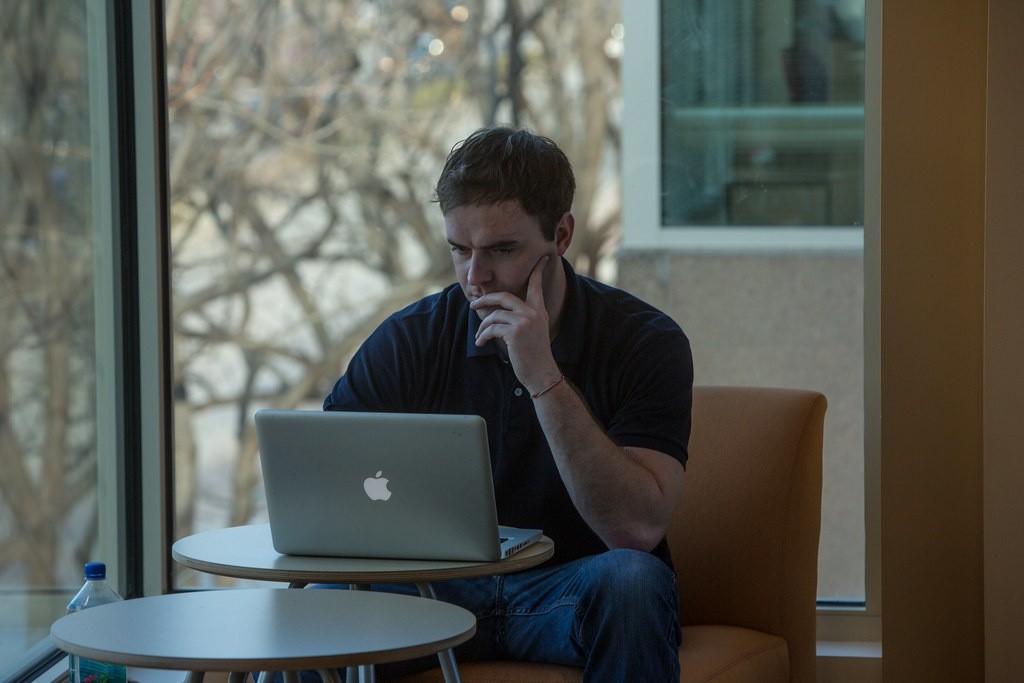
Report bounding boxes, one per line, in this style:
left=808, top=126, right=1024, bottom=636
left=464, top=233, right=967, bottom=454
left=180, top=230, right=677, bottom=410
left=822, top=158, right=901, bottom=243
left=324, top=125, right=694, bottom=683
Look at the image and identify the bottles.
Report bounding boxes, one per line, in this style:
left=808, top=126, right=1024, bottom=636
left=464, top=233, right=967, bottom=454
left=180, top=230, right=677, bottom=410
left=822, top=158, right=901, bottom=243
left=65, top=562, right=128, bottom=683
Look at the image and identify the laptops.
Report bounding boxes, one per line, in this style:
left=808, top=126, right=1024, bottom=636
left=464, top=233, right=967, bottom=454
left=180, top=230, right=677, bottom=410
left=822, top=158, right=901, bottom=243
left=253, top=410, right=543, bottom=563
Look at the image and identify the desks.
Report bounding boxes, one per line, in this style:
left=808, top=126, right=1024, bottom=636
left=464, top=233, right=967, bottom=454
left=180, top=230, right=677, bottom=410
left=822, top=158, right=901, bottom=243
left=46, top=520, right=558, bottom=683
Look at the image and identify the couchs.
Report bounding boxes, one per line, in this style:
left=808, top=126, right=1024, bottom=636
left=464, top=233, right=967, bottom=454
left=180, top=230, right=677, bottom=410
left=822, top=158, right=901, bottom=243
left=411, top=383, right=828, bottom=683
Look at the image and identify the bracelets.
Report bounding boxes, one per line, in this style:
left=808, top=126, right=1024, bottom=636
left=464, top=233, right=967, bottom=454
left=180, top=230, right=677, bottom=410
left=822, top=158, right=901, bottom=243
left=530, top=376, right=565, bottom=399
